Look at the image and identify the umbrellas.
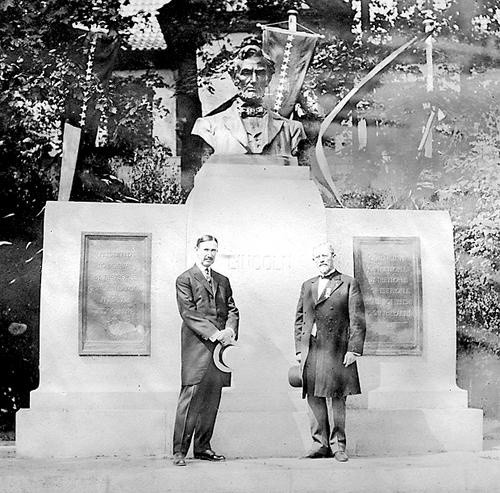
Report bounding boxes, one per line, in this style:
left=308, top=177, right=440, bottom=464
left=195, top=454, right=225, bottom=462
left=303, top=451, right=332, bottom=458
left=335, top=451, right=348, bottom=462
left=173, top=458, right=186, bottom=465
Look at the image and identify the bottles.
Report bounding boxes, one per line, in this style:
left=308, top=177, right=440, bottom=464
left=289, top=363, right=304, bottom=388
left=213, top=338, right=245, bottom=373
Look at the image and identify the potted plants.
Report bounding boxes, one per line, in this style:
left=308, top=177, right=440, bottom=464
left=77, top=228, right=155, bottom=358
left=349, top=232, right=425, bottom=358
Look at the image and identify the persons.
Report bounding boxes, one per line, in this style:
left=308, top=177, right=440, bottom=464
left=294, top=242, right=366, bottom=463
left=170, top=235, right=240, bottom=466
left=191, top=48, right=308, bottom=156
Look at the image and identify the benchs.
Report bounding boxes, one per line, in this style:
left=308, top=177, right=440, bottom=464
left=260, top=25, right=319, bottom=122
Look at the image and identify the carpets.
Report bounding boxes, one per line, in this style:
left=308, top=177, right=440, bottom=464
left=205, top=269, right=213, bottom=291
